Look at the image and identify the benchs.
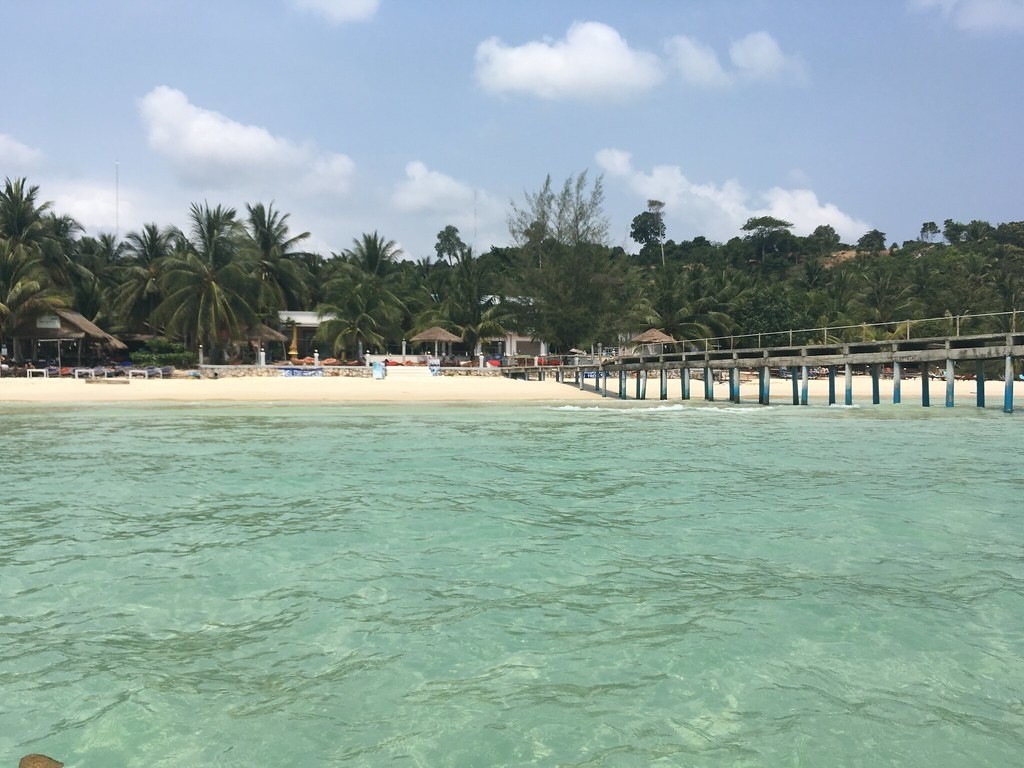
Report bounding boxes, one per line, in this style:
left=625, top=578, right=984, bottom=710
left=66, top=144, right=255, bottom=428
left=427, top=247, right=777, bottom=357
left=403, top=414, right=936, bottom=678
left=75, top=370, right=96, bottom=378
left=129, top=370, right=148, bottom=378
left=27, top=369, right=49, bottom=379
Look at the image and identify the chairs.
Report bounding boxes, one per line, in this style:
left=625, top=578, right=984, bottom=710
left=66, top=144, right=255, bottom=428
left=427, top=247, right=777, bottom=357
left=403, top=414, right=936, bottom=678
left=74, top=365, right=122, bottom=379
left=128, top=364, right=175, bottom=380
left=41, top=367, right=73, bottom=378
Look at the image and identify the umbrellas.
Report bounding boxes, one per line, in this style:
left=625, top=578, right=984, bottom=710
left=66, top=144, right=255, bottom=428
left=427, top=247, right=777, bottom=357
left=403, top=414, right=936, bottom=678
left=633, top=328, right=677, bottom=354
left=223, top=323, right=288, bottom=364
left=410, top=327, right=463, bottom=358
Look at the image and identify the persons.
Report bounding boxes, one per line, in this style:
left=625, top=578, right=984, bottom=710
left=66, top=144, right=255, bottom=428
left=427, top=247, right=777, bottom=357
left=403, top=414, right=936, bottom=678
left=442, top=352, right=450, bottom=362
